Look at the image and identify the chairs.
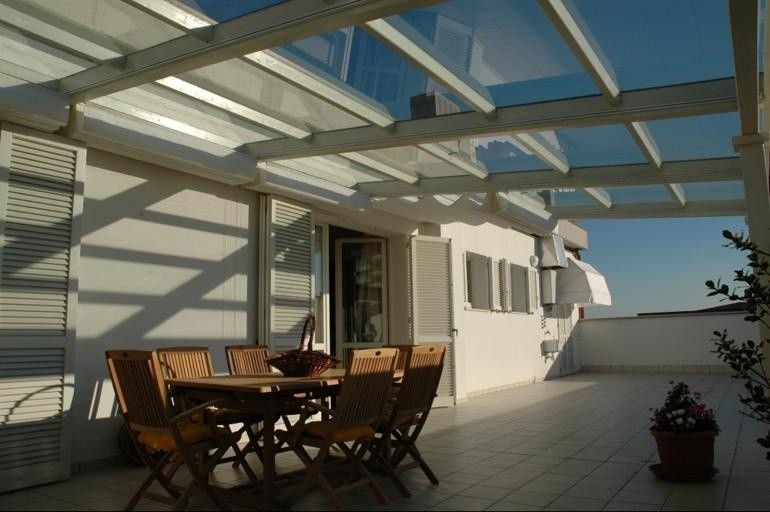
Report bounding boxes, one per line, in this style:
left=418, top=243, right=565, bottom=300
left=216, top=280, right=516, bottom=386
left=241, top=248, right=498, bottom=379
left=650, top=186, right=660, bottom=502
left=104, top=342, right=450, bottom=510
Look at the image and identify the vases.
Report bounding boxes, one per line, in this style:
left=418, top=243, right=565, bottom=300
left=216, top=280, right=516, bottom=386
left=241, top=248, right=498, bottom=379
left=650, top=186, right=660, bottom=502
left=650, top=423, right=719, bottom=482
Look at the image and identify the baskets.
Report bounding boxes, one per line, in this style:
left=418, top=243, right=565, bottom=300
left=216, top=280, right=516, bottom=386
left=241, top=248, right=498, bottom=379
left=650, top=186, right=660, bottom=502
left=266, top=315, right=342, bottom=378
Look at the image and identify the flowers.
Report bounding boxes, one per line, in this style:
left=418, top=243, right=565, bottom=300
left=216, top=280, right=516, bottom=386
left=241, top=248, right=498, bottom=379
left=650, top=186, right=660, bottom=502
left=651, top=380, right=721, bottom=435
